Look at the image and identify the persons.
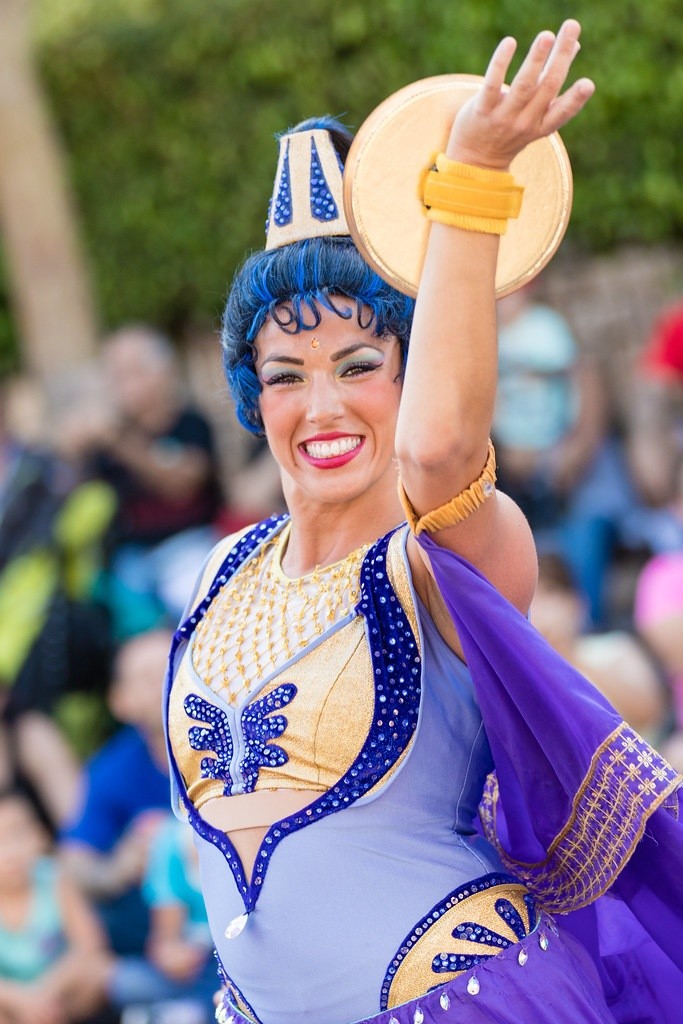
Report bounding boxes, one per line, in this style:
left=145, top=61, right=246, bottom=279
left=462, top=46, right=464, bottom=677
left=161, top=19, right=683, bottom=1024
left=0, top=245, right=683, bottom=1024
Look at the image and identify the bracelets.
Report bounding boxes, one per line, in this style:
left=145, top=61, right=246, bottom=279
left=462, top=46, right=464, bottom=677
left=424, top=153, right=514, bottom=236
